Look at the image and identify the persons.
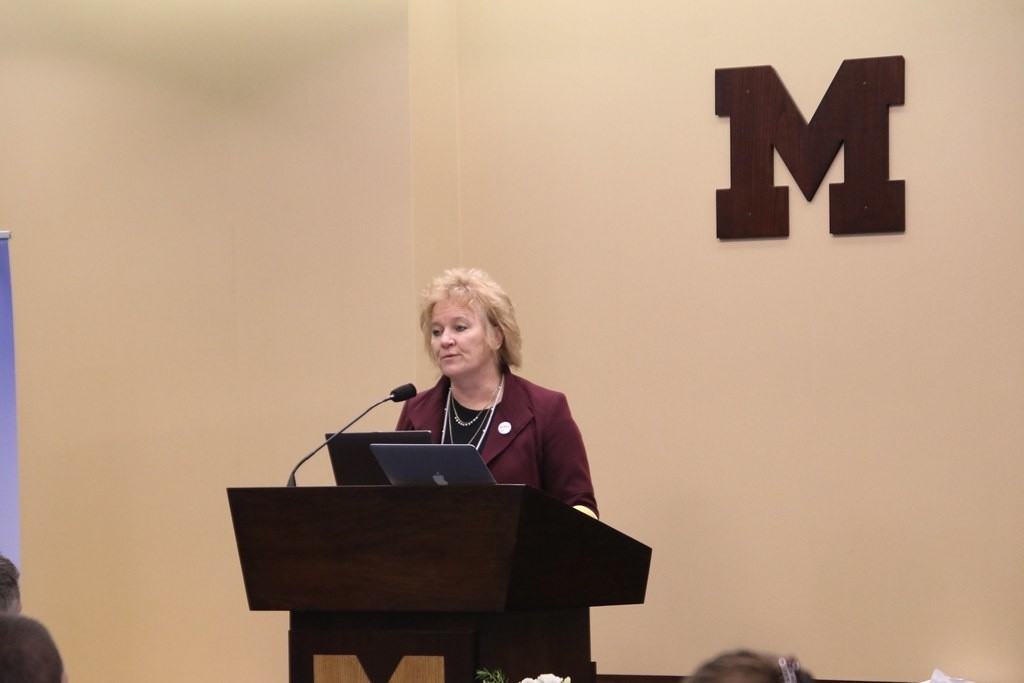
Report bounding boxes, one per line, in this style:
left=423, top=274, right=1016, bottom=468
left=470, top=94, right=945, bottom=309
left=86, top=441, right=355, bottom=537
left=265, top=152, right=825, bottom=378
left=394, top=267, right=600, bottom=522
left=688, top=648, right=819, bottom=682
left=0, top=555, right=69, bottom=683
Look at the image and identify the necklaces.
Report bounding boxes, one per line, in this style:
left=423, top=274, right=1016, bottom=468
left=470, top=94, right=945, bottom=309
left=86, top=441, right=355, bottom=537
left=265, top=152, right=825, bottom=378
left=441, top=372, right=505, bottom=450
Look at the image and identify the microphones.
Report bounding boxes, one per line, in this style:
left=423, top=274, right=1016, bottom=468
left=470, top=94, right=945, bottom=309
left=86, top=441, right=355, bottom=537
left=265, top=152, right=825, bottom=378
left=287, top=385, right=417, bottom=486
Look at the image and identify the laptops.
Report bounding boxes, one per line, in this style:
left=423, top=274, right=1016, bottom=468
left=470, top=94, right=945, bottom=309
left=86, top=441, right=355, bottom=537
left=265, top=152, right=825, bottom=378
left=369, top=441, right=496, bottom=486
left=326, top=430, right=432, bottom=486
